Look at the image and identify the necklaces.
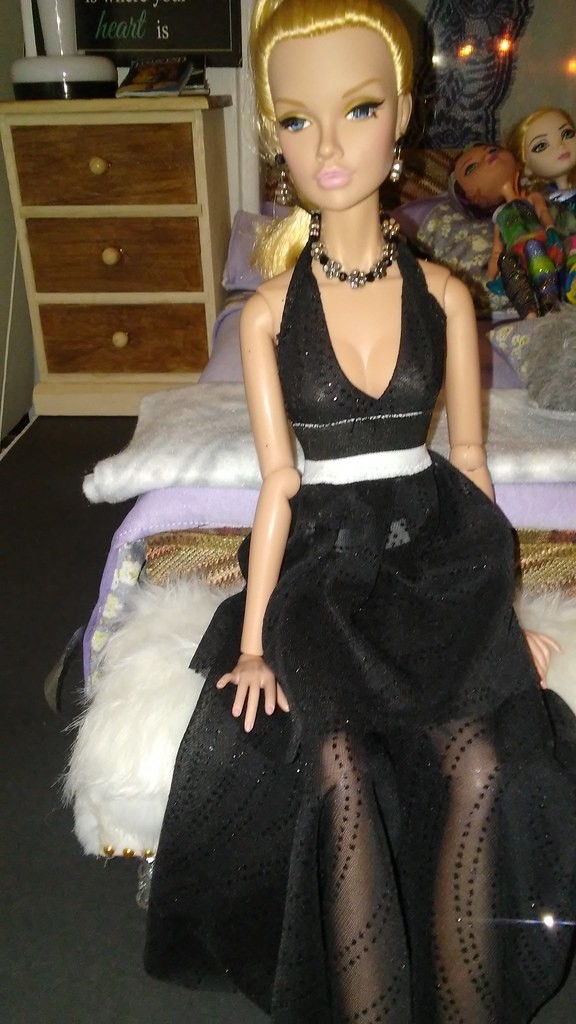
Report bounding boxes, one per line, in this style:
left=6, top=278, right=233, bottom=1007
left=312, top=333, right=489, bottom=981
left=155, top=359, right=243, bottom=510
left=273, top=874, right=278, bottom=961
left=304, top=203, right=401, bottom=286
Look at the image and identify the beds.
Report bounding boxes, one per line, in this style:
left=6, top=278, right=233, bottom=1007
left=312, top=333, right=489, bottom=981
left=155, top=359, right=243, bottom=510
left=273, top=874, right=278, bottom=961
left=67, top=194, right=576, bottom=892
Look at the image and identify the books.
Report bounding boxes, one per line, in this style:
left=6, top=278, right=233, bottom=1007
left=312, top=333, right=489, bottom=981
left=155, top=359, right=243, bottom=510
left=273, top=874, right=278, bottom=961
left=116, top=49, right=210, bottom=97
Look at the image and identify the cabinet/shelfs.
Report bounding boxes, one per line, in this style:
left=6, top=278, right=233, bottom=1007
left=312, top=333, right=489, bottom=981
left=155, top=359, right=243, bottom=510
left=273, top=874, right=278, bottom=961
left=1, top=95, right=238, bottom=418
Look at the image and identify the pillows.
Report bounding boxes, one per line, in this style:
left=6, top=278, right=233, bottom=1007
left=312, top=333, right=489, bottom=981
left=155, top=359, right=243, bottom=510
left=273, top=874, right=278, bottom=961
left=388, top=196, right=526, bottom=326
left=481, top=310, right=576, bottom=422
left=220, top=206, right=273, bottom=291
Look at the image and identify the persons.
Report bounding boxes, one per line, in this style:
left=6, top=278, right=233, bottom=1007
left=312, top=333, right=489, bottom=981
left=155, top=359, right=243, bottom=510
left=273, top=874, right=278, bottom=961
left=508, top=105, right=576, bottom=304
left=449, top=141, right=560, bottom=319
left=141, top=1, right=576, bottom=1024
